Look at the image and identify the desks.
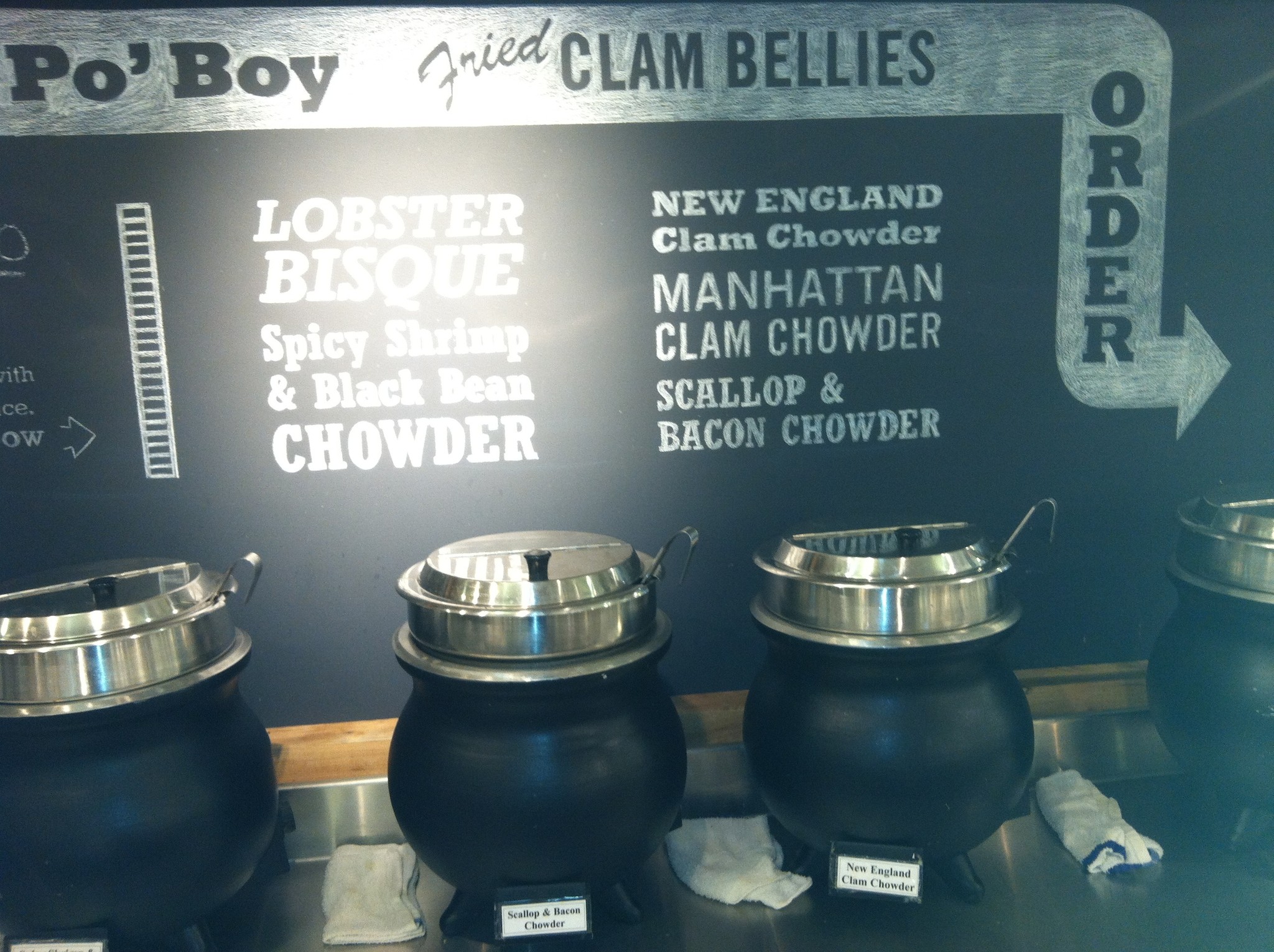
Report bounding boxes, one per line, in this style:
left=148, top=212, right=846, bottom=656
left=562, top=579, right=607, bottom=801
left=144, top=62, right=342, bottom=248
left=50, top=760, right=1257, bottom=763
left=205, top=767, right=1274, bottom=952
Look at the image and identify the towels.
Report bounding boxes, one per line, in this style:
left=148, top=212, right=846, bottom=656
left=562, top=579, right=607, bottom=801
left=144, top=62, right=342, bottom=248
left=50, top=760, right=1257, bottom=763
left=666, top=813, right=813, bottom=910
left=1035, top=768, right=1164, bottom=876
left=321, top=840, right=424, bottom=943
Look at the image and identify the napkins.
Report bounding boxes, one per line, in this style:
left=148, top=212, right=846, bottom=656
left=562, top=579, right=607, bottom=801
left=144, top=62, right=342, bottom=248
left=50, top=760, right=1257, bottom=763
left=660, top=810, right=816, bottom=911
left=1031, top=766, right=1168, bottom=876
left=321, top=841, right=427, bottom=946
